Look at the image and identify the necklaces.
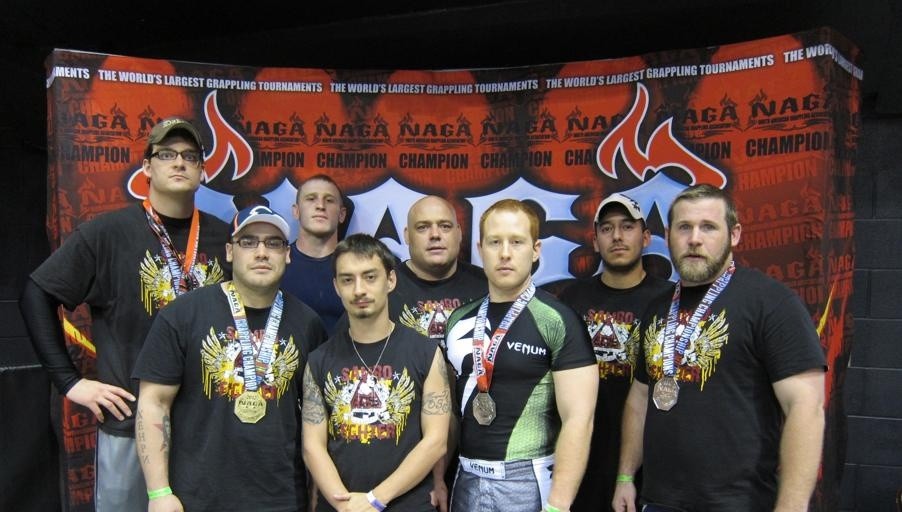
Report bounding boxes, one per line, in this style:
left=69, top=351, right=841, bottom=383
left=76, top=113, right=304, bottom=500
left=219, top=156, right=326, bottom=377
left=348, top=327, right=393, bottom=387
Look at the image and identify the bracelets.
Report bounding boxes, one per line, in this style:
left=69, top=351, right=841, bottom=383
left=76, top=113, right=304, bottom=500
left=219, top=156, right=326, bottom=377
left=545, top=503, right=561, bottom=512
left=616, top=474, right=635, bottom=482
left=147, top=487, right=173, bottom=500
left=366, top=491, right=386, bottom=511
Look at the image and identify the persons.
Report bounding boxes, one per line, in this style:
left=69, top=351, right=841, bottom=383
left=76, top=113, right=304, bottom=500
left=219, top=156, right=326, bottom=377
left=332, top=192, right=490, bottom=405
left=18, top=119, right=232, bottom=512
left=134, top=203, right=331, bottom=512
left=301, top=233, right=454, bottom=512
left=555, top=192, right=678, bottom=512
left=611, top=183, right=829, bottom=512
left=277, top=173, right=348, bottom=339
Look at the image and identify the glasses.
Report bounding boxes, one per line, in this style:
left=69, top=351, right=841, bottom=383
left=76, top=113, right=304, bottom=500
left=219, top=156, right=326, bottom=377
left=149, top=148, right=200, bottom=163
left=231, top=234, right=289, bottom=249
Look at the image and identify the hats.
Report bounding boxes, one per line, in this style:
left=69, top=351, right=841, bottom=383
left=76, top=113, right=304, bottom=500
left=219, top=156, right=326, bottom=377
left=227, top=203, right=291, bottom=242
left=593, top=191, right=647, bottom=233
left=144, top=117, right=203, bottom=159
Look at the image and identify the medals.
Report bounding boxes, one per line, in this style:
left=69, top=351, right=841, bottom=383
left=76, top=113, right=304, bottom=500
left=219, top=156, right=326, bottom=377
left=433, top=198, right=598, bottom=512
left=651, top=377, right=679, bottom=412
left=234, top=390, right=267, bottom=423
left=471, top=390, right=497, bottom=427
